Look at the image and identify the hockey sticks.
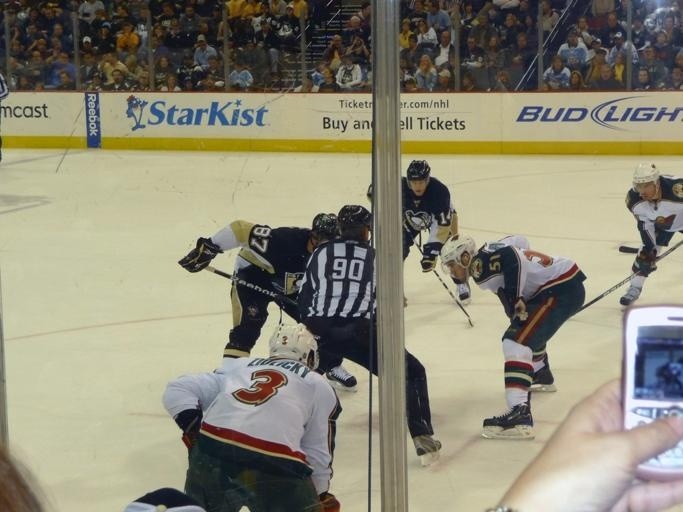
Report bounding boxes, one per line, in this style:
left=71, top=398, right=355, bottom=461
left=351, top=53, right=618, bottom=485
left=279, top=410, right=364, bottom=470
left=402, top=223, right=474, bottom=329
left=619, top=245, right=639, bottom=255
left=572, top=237, right=683, bottom=316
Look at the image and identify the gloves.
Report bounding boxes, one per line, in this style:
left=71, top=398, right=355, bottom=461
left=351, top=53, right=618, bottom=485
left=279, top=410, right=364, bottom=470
left=180, top=236, right=219, bottom=272
left=638, top=250, right=657, bottom=277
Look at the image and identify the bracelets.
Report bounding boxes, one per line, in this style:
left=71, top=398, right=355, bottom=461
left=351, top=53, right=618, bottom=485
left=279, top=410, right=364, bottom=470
left=486, top=506, right=516, bottom=512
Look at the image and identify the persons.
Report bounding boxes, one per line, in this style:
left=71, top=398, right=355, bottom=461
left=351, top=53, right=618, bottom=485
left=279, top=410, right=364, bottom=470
left=442, top=233, right=586, bottom=436
left=1, top=1, right=373, bottom=93
left=399, top=1, right=682, bottom=91
left=163, top=324, right=342, bottom=512
left=294, top=204, right=442, bottom=457
left=368, top=159, right=471, bottom=303
left=0, top=72, right=9, bottom=160
left=486, top=377, right=683, bottom=512
left=619, top=160, right=683, bottom=305
left=179, top=213, right=359, bottom=392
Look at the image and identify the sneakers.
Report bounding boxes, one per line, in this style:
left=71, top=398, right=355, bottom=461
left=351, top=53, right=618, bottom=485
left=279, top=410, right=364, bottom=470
left=326, top=367, right=357, bottom=386
left=484, top=403, right=533, bottom=427
left=412, top=435, right=442, bottom=456
left=458, top=284, right=470, bottom=300
left=621, top=286, right=642, bottom=305
left=531, top=367, right=556, bottom=384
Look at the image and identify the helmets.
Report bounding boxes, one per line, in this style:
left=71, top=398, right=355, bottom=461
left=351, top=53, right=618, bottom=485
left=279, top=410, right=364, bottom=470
left=268, top=323, right=319, bottom=370
left=314, top=160, right=477, bottom=281
left=632, top=163, right=660, bottom=183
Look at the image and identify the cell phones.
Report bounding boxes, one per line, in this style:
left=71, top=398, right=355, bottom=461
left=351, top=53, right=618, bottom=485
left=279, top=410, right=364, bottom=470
left=621, top=303, right=682, bottom=482
